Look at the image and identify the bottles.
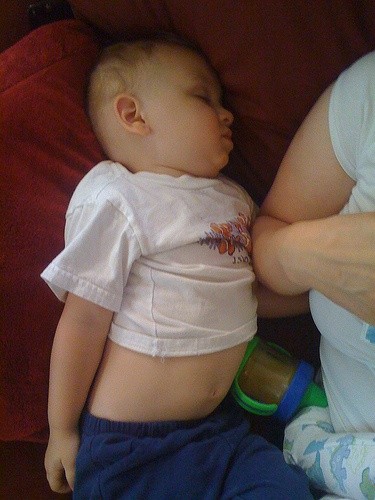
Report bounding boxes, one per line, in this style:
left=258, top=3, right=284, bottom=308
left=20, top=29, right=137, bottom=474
left=232, top=336, right=328, bottom=425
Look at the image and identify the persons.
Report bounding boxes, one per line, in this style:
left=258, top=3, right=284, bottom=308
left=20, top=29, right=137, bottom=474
left=250, top=45, right=375, bottom=499
left=43, top=17, right=316, bottom=500
